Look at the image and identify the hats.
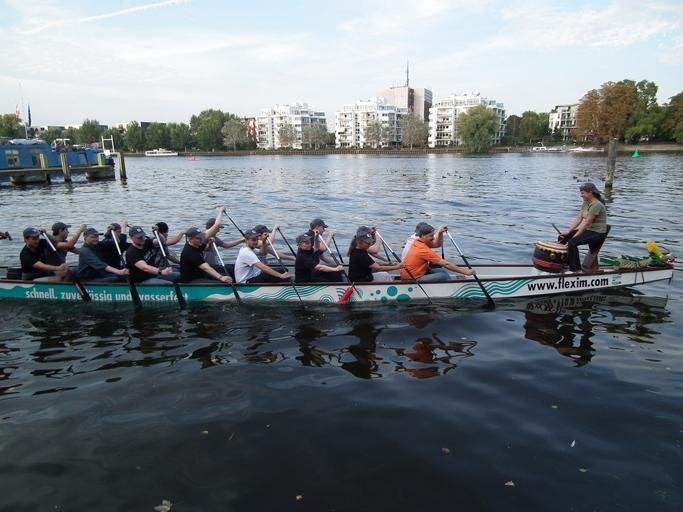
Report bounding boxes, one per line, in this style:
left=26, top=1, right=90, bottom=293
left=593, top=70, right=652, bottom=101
left=206, top=217, right=224, bottom=228
left=22, top=228, right=40, bottom=237
left=578, top=183, right=601, bottom=194
left=51, top=222, right=71, bottom=230
left=244, top=219, right=329, bottom=243
left=128, top=226, right=144, bottom=238
left=187, top=227, right=205, bottom=238
left=82, top=227, right=103, bottom=236
left=104, top=223, right=120, bottom=238
left=356, top=222, right=434, bottom=244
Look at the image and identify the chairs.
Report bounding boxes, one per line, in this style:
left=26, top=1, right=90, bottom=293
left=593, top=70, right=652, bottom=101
left=572, top=223, right=614, bottom=273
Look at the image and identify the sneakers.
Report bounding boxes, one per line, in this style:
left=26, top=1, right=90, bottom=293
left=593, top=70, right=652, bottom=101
left=564, top=270, right=581, bottom=274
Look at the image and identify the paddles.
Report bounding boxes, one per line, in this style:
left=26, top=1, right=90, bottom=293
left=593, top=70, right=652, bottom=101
left=154, top=229, right=188, bottom=308
left=111, top=229, right=143, bottom=306
left=43, top=232, right=91, bottom=302
left=444, top=228, right=497, bottom=308
left=212, top=241, right=243, bottom=305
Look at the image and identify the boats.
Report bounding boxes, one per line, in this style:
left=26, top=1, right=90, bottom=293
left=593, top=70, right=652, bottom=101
left=526, top=145, right=606, bottom=154
left=0, top=118, right=116, bottom=169
left=144, top=146, right=180, bottom=157
left=0, top=251, right=676, bottom=309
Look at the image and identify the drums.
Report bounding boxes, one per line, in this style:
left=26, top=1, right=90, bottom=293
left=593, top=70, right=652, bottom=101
left=532, top=241, right=569, bottom=272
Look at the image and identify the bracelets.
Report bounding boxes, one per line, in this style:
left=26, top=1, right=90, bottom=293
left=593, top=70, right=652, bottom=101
left=158, top=270, right=162, bottom=275
left=217, top=274, right=222, bottom=279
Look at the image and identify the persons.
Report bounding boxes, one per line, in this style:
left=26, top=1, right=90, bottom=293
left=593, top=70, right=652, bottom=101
left=19, top=228, right=71, bottom=282
left=47, top=222, right=86, bottom=281
left=79, top=225, right=130, bottom=283
left=558, top=182, right=606, bottom=274
left=151, top=222, right=186, bottom=271
left=126, top=225, right=181, bottom=285
left=179, top=227, right=234, bottom=283
left=234, top=218, right=476, bottom=284
left=201, top=207, right=245, bottom=276
left=102, top=219, right=133, bottom=270
